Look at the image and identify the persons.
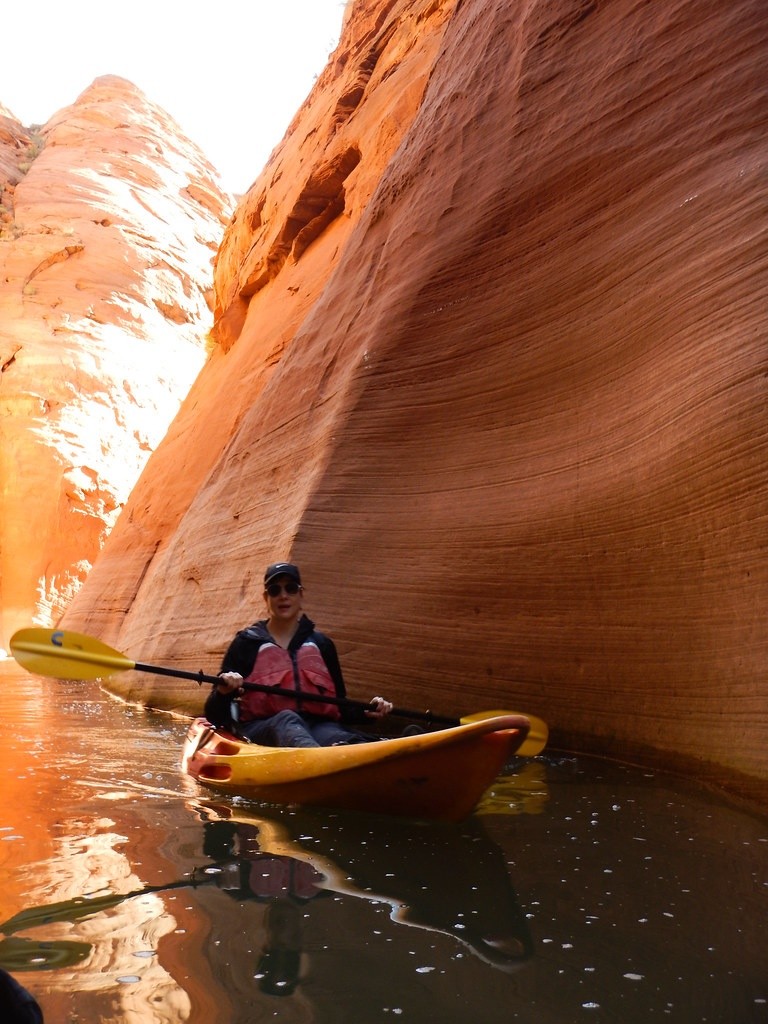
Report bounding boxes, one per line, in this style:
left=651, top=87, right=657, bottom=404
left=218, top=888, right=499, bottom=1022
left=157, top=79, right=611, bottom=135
left=203, top=563, right=393, bottom=748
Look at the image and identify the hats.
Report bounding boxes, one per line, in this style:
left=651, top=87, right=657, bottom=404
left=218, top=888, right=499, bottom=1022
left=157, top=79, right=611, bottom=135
left=264, top=563, right=301, bottom=586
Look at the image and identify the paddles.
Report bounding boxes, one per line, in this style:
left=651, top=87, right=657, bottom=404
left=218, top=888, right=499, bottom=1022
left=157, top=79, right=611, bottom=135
left=10, top=627, right=546, bottom=760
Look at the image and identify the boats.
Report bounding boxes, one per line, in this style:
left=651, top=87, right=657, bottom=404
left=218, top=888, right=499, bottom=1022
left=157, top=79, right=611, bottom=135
left=178, top=715, right=535, bottom=825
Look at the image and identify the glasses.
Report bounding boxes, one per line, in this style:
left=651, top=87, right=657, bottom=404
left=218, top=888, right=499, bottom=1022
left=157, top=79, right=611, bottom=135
left=267, top=583, right=300, bottom=597
